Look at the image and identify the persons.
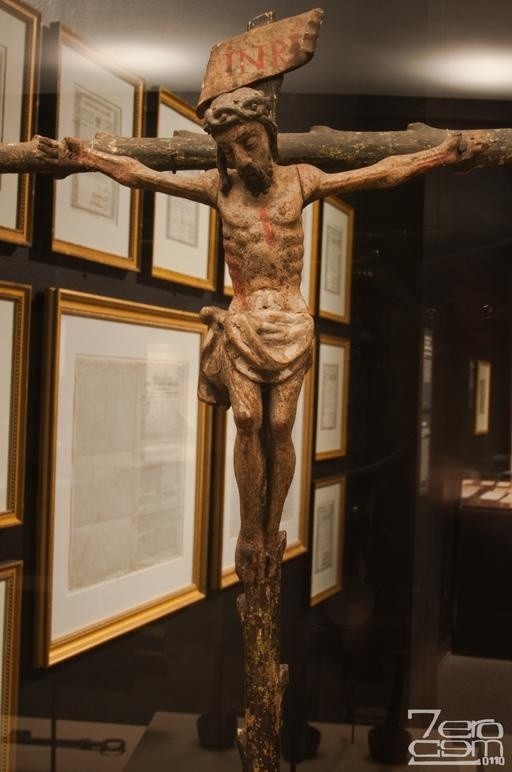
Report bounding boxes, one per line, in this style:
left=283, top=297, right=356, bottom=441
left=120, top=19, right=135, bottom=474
left=28, top=85, right=483, bottom=580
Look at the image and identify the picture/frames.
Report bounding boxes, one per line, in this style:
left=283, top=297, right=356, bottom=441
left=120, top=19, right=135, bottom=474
left=317, top=195, right=355, bottom=325
left=314, top=333, right=355, bottom=464
left=464, top=357, right=494, bottom=440
left=214, top=175, right=319, bottom=318
left=304, top=475, right=346, bottom=608
left=0, top=0, right=42, bottom=250
left=209, top=362, right=314, bottom=596
left=43, top=21, right=147, bottom=274
left=142, top=86, right=220, bottom=295
left=0, top=280, right=34, bottom=772
left=33, top=286, right=209, bottom=672
